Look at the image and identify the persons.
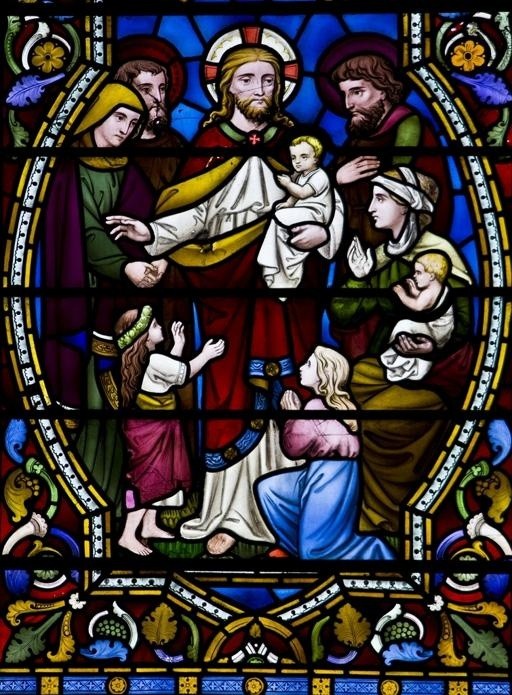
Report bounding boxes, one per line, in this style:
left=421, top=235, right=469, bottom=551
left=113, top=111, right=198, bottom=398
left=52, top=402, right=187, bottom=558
left=114, top=303, right=226, bottom=559
left=101, top=29, right=349, bottom=559
left=312, top=31, right=457, bottom=360
left=256, top=134, right=344, bottom=304
left=382, top=250, right=454, bottom=381
left=109, top=33, right=202, bottom=495
left=39, top=80, right=168, bottom=519
left=344, top=163, right=475, bottom=534
left=252, top=344, right=397, bottom=559
